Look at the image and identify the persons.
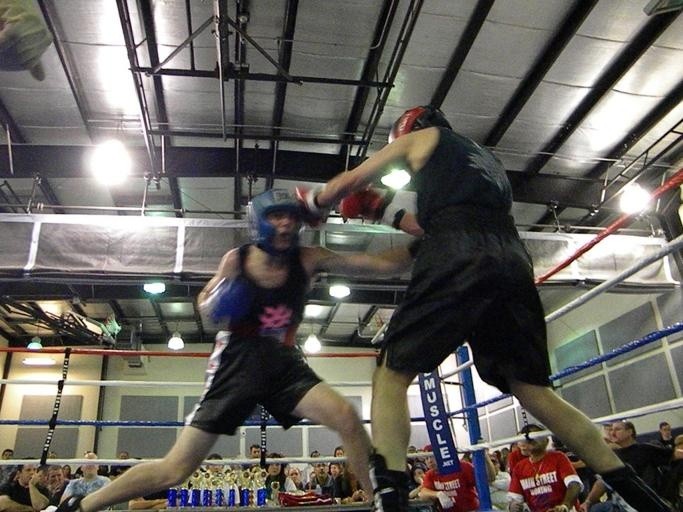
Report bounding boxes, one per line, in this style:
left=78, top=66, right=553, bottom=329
left=40, top=464, right=71, bottom=507
left=480, top=454, right=511, bottom=492
left=0, top=457, right=50, bottom=512
left=244, top=445, right=264, bottom=470
left=294, top=106, right=675, bottom=511
left=34, top=465, right=48, bottom=492
left=184, top=454, right=240, bottom=506
left=333, top=446, right=344, bottom=465
left=0, top=4, right=53, bottom=82
left=328, top=463, right=344, bottom=483
left=264, top=453, right=297, bottom=504
left=337, top=463, right=369, bottom=507
left=508, top=423, right=583, bottom=511
left=495, top=439, right=521, bottom=471
left=306, top=451, right=319, bottom=481
left=111, top=450, right=130, bottom=476
left=0, top=448, right=15, bottom=485
left=408, top=466, right=425, bottom=500
left=601, top=421, right=617, bottom=444
left=286, top=467, right=305, bottom=495
left=128, top=490, right=182, bottom=511
left=654, top=421, right=683, bottom=455
left=62, top=453, right=113, bottom=511
left=576, top=422, right=682, bottom=512
left=408, top=445, right=426, bottom=468
left=309, top=460, right=335, bottom=497
left=62, top=465, right=71, bottom=477
left=419, top=441, right=496, bottom=511
left=56, top=189, right=413, bottom=512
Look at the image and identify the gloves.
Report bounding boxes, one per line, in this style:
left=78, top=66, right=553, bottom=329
left=295, top=185, right=330, bottom=228
left=337, top=182, right=387, bottom=224
left=200, top=277, right=258, bottom=324
left=436, top=491, right=454, bottom=509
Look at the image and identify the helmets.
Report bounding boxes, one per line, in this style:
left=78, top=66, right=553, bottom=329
left=389, top=106, right=454, bottom=148
left=247, top=189, right=305, bottom=250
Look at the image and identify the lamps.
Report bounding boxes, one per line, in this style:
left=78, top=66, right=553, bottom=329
left=168, top=332, right=185, bottom=350
left=305, top=333, right=321, bottom=354
left=28, top=337, right=42, bottom=349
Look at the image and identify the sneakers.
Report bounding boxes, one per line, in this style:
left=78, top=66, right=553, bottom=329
left=55, top=494, right=84, bottom=512
left=368, top=447, right=421, bottom=512
left=593, top=463, right=679, bottom=512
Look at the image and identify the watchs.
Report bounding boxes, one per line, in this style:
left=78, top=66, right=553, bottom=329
left=584, top=499, right=593, bottom=505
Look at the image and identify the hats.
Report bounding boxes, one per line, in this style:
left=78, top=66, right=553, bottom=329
left=521, top=423, right=544, bottom=432
